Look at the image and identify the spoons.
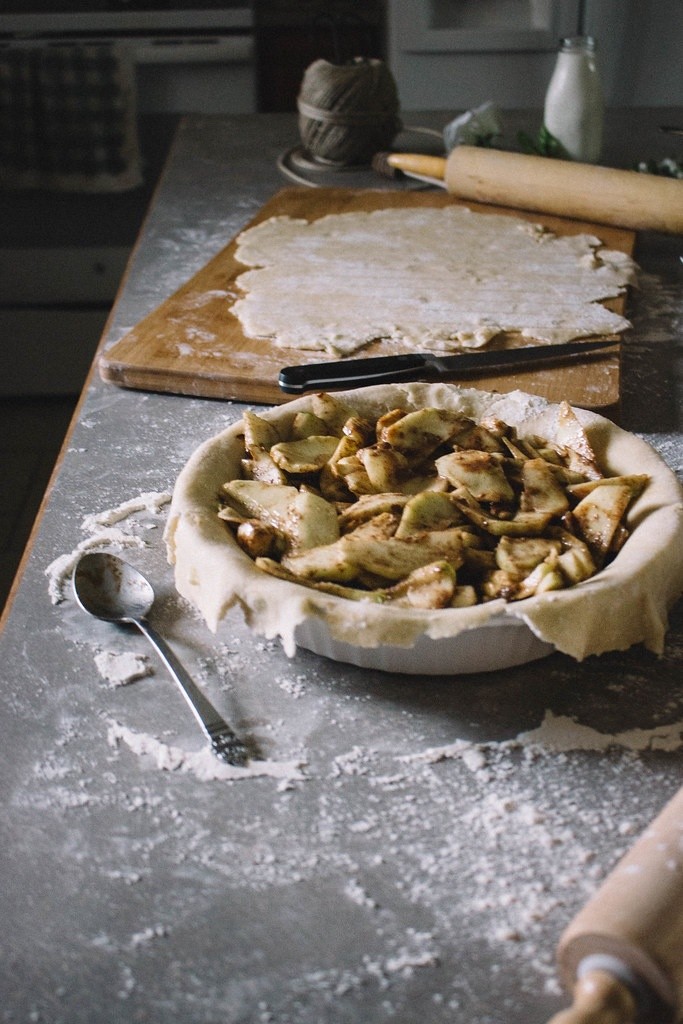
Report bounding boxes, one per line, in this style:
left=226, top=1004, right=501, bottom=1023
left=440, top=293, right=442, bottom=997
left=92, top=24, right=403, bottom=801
left=73, top=553, right=257, bottom=767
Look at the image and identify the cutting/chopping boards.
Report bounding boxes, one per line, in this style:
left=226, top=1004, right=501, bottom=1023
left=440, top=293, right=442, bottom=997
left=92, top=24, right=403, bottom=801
left=99, top=186, right=636, bottom=406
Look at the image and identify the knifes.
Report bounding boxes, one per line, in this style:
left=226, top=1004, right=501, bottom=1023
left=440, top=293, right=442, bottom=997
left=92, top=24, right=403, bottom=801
left=277, top=340, right=621, bottom=396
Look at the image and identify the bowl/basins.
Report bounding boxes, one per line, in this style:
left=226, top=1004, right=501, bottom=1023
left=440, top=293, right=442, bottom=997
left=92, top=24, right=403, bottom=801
left=162, top=383, right=682, bottom=674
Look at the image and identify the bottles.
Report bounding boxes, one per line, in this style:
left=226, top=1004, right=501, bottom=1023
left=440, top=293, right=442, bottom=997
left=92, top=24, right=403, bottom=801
left=535, top=34, right=605, bottom=167
